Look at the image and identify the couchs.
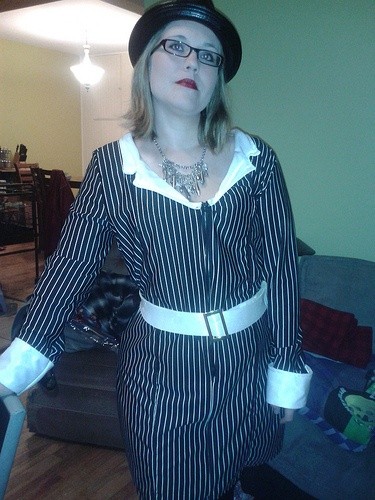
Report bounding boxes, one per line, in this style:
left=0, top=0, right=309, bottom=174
left=11, top=237, right=375, bottom=500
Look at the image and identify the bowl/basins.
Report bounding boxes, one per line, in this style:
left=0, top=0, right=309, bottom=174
left=0, top=160, right=13, bottom=169
left=0, top=150, right=12, bottom=160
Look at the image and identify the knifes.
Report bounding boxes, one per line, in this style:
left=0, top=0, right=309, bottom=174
left=16, top=144, right=27, bottom=162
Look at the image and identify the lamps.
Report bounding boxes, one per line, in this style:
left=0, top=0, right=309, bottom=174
left=70, top=44, right=105, bottom=93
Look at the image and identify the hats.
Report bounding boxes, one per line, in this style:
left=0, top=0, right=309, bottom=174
left=128, top=0, right=242, bottom=84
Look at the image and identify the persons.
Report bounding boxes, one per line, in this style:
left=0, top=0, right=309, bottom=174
left=0, top=0, right=314, bottom=500
left=0, top=289, right=17, bottom=317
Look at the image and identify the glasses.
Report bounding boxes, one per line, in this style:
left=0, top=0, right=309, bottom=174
left=149, top=39, right=223, bottom=75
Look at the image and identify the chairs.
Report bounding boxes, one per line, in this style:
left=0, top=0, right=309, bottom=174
left=30, top=167, right=75, bottom=284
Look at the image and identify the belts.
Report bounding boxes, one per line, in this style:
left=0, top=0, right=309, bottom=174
left=139, top=280, right=269, bottom=340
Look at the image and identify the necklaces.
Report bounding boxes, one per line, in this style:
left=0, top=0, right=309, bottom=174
left=153, top=136, right=209, bottom=204
